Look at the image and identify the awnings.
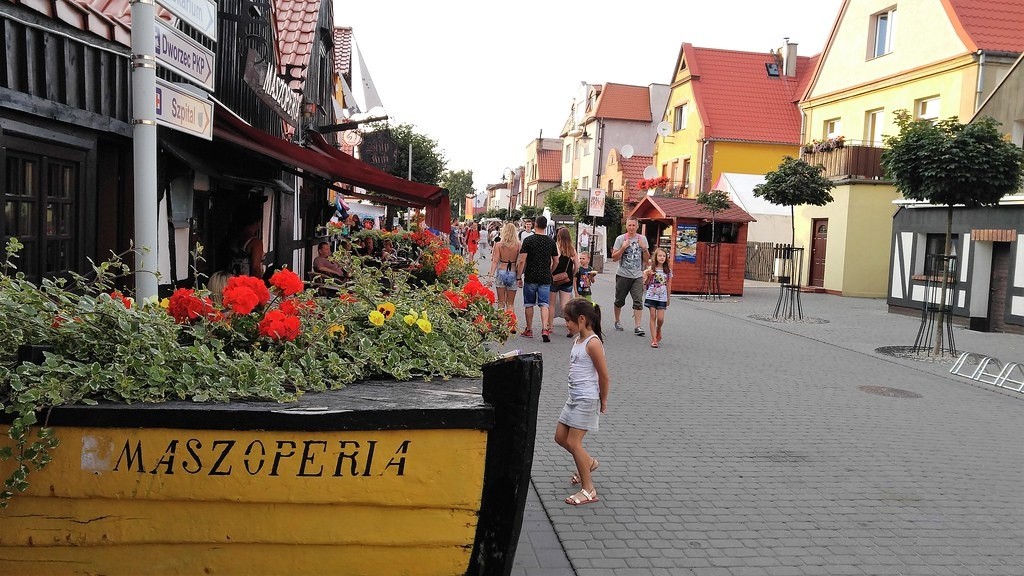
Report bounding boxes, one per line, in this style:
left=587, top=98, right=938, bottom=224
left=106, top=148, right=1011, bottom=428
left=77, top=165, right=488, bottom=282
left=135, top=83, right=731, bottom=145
left=204, top=90, right=451, bottom=234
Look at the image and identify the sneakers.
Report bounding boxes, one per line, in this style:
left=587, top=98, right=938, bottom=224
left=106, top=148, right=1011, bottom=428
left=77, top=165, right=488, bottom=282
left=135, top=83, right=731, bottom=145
left=613, top=321, right=623, bottom=331
left=542, top=329, right=550, bottom=342
left=634, top=326, right=646, bottom=334
left=521, top=327, right=533, bottom=337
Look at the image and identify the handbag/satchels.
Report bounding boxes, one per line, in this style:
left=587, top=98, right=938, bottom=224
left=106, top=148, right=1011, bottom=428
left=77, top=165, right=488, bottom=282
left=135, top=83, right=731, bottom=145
left=553, top=272, right=570, bottom=286
left=229, top=236, right=263, bottom=277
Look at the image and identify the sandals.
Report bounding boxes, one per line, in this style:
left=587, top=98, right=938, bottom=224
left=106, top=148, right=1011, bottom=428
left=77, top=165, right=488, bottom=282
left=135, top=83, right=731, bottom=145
left=656, top=329, right=663, bottom=342
left=565, top=489, right=599, bottom=505
left=651, top=338, right=658, bottom=347
left=570, top=459, right=599, bottom=484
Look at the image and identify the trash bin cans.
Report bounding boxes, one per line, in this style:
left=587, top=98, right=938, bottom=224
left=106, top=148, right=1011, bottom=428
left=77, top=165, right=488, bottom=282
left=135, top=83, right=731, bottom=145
left=593, top=252, right=605, bottom=274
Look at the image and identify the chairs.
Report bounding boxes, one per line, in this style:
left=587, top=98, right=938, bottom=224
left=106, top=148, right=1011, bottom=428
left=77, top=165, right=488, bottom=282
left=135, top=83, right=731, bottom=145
left=312, top=265, right=344, bottom=281
left=308, top=272, right=343, bottom=285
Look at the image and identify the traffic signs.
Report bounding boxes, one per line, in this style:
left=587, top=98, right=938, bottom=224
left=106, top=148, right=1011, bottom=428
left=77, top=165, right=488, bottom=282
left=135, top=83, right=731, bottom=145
left=155, top=0, right=217, bottom=142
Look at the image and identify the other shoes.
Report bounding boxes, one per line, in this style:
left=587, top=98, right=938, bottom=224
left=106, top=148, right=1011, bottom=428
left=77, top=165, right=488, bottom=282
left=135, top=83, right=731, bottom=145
left=548, top=327, right=554, bottom=333
left=566, top=330, right=572, bottom=337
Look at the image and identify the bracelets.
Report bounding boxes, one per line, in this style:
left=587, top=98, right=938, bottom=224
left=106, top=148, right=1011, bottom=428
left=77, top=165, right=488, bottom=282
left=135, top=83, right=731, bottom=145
left=516, top=278, right=521, bottom=281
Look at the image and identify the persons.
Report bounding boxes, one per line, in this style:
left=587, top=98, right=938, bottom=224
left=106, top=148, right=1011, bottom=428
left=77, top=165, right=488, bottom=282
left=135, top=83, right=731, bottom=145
left=516, top=216, right=559, bottom=342
left=612, top=217, right=650, bottom=335
left=644, top=248, right=673, bottom=348
left=554, top=299, right=609, bottom=505
left=573, top=251, right=595, bottom=301
left=517, top=220, right=534, bottom=240
left=487, top=223, right=522, bottom=334
left=207, top=249, right=250, bottom=303
left=451, top=218, right=501, bottom=265
left=226, top=201, right=263, bottom=278
left=580, top=229, right=591, bottom=252
left=314, top=214, right=397, bottom=276
left=548, top=228, right=579, bottom=338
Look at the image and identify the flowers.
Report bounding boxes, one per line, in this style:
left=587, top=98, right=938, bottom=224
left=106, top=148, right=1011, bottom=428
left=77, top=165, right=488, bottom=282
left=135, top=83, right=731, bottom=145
left=798, top=132, right=846, bottom=157
left=636, top=176, right=670, bottom=190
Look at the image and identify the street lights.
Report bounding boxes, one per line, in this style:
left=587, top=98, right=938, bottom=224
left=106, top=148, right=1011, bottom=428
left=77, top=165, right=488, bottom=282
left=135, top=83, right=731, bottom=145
left=500, top=166, right=515, bottom=220
left=577, top=116, right=605, bottom=264
